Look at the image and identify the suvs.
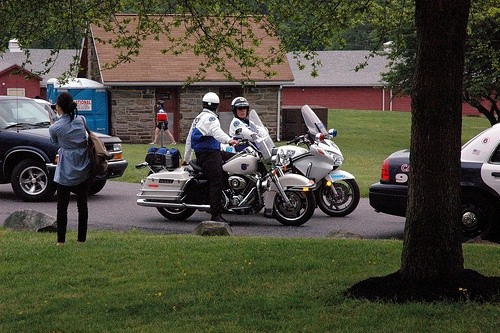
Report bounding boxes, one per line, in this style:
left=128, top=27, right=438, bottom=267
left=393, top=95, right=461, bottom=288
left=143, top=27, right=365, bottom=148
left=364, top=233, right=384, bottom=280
left=0, top=95, right=129, bottom=201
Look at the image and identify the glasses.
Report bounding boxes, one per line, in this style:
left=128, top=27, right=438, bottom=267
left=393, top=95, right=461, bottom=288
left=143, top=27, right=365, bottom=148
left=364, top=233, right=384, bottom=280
left=237, top=107, right=247, bottom=110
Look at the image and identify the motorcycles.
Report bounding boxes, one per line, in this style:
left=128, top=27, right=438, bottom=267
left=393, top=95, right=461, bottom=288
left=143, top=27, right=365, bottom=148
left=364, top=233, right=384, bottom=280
left=134, top=110, right=318, bottom=227
left=244, top=104, right=362, bottom=217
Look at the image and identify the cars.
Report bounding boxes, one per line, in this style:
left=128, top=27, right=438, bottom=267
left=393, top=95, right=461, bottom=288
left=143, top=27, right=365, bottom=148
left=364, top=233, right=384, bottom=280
left=367, top=124, right=500, bottom=243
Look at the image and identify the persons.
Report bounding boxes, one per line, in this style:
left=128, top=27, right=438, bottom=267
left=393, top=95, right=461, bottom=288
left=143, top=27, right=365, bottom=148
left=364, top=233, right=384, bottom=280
left=49, top=92, right=91, bottom=246
left=149, top=100, right=177, bottom=145
left=181, top=92, right=239, bottom=227
left=229, top=97, right=264, bottom=215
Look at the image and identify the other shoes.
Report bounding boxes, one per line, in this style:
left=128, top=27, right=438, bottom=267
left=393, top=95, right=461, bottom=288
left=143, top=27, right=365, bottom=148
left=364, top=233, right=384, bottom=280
left=211, top=216, right=232, bottom=226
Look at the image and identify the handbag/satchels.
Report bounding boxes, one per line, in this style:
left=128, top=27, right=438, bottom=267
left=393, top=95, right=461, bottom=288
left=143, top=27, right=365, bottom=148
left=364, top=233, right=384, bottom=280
left=80, top=116, right=108, bottom=175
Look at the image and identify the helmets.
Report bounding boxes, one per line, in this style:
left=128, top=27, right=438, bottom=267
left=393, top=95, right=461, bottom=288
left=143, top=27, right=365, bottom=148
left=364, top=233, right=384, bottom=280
left=231, top=96, right=249, bottom=112
left=202, top=92, right=219, bottom=106
